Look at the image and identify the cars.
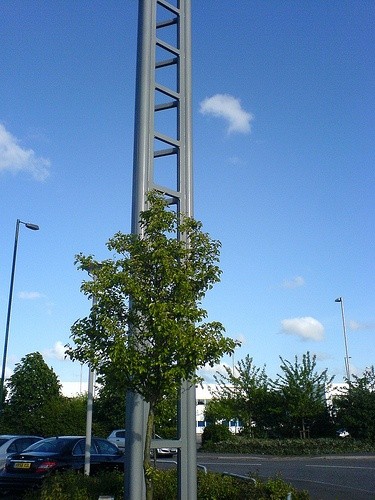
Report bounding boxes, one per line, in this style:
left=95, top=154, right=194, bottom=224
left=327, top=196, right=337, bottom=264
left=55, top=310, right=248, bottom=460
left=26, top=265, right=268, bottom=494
left=107, top=429, right=178, bottom=460
left=0, top=435, right=125, bottom=498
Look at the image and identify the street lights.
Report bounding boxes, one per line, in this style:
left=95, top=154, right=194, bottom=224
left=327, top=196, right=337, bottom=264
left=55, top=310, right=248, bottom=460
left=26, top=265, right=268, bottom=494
left=334, top=296, right=351, bottom=381
left=0, top=219, right=40, bottom=406
